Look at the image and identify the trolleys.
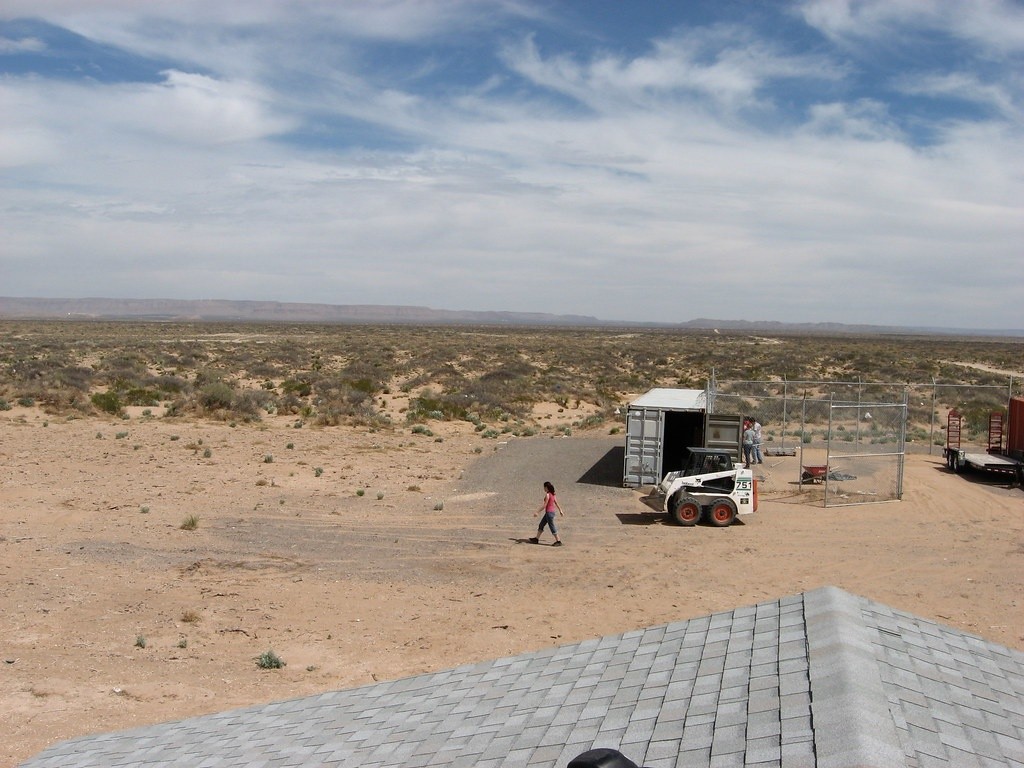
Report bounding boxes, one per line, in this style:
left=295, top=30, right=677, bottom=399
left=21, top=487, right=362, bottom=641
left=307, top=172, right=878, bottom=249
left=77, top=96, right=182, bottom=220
left=802, top=464, right=840, bottom=484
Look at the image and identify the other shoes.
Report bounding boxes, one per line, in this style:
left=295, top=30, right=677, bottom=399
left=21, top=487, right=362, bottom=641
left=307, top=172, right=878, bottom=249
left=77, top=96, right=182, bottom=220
left=552, top=541, right=562, bottom=546
left=529, top=537, right=539, bottom=544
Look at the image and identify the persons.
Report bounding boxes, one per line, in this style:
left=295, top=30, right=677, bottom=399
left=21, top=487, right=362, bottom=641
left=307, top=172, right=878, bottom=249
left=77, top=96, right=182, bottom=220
left=743, top=417, right=763, bottom=468
left=530, top=482, right=563, bottom=547
